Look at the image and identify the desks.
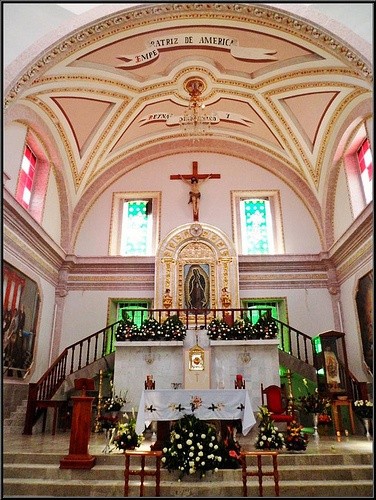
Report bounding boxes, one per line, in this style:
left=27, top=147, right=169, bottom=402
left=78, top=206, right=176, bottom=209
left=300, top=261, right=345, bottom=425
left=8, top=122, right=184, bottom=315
left=34, top=400, right=69, bottom=436
left=324, top=399, right=354, bottom=442
left=134, top=389, right=256, bottom=440
left=123, top=451, right=278, bottom=497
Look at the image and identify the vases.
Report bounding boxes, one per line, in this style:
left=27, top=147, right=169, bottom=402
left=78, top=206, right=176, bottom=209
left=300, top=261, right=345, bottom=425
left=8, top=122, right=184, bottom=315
left=312, top=413, right=319, bottom=436
left=362, top=418, right=370, bottom=437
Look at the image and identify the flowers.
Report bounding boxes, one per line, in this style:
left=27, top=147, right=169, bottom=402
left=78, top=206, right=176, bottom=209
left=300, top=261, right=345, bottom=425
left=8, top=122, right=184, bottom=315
left=158, top=414, right=227, bottom=483
left=115, top=312, right=279, bottom=340
left=254, top=407, right=285, bottom=453
left=353, top=400, right=374, bottom=420
left=285, top=420, right=309, bottom=452
left=99, top=381, right=145, bottom=454
left=292, top=378, right=332, bottom=416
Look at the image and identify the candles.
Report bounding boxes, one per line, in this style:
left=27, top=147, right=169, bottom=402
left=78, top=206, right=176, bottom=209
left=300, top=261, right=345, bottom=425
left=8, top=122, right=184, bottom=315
left=236, top=375, right=243, bottom=382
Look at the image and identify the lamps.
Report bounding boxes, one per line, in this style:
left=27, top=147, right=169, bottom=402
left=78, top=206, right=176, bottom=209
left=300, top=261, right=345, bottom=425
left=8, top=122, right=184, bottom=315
left=180, top=80, right=211, bottom=144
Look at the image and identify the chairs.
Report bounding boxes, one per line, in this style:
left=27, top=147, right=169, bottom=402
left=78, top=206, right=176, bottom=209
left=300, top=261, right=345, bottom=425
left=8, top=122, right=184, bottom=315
left=261, top=383, right=293, bottom=427
